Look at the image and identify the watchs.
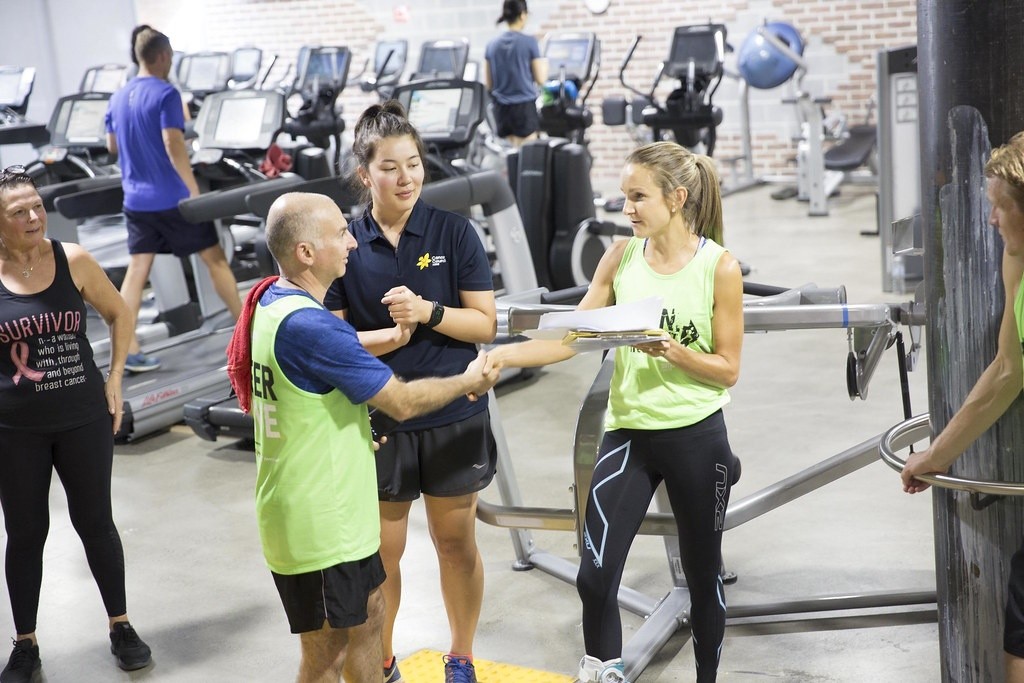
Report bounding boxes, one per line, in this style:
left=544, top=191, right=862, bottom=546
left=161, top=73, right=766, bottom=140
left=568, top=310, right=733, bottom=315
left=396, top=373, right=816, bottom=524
left=422, top=301, right=444, bottom=329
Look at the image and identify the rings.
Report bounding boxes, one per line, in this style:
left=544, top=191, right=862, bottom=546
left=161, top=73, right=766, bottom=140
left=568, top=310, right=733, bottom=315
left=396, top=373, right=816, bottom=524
left=648, top=348, right=652, bottom=352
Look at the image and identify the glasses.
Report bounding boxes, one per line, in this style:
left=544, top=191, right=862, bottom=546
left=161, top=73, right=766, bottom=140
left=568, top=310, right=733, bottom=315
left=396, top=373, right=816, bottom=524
left=0, top=165, right=27, bottom=182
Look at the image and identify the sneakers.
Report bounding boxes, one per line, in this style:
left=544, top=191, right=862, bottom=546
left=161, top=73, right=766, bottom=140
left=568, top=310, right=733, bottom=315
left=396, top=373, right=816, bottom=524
left=0, top=636, right=43, bottom=683
left=384, top=655, right=404, bottom=683
left=110, top=621, right=152, bottom=669
left=442, top=654, right=476, bottom=683
left=124, top=350, right=161, bottom=371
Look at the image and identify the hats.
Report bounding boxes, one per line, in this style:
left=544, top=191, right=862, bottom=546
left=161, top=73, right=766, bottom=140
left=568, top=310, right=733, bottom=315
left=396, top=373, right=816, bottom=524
left=496, top=0, right=527, bottom=23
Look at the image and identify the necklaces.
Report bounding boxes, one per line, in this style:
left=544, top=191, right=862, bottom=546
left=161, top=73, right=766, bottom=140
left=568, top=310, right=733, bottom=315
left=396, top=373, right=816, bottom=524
left=22, top=257, right=41, bottom=278
left=281, top=275, right=307, bottom=292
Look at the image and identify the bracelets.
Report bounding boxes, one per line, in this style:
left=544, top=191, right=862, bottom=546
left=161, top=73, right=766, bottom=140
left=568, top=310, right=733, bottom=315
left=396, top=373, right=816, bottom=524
left=106, top=370, right=123, bottom=377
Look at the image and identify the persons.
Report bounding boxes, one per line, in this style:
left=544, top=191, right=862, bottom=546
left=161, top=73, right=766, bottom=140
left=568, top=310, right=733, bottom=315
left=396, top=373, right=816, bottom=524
left=466, top=142, right=744, bottom=683
left=125, top=25, right=191, bottom=124
left=104, top=28, right=244, bottom=371
left=248, top=192, right=504, bottom=683
left=0, top=164, right=151, bottom=683
left=486, top=0, right=548, bottom=149
left=325, top=105, right=498, bottom=683
left=901, top=133, right=1024, bottom=683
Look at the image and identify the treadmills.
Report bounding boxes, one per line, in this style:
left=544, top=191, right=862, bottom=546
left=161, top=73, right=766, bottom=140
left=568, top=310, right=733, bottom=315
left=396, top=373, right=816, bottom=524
left=0, top=21, right=732, bottom=453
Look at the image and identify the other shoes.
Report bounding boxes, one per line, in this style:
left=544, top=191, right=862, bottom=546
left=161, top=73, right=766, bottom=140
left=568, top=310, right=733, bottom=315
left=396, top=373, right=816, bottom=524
left=573, top=655, right=626, bottom=683
